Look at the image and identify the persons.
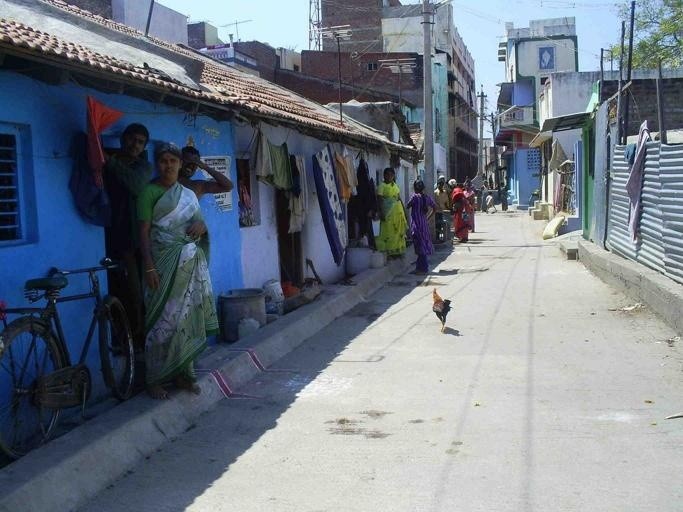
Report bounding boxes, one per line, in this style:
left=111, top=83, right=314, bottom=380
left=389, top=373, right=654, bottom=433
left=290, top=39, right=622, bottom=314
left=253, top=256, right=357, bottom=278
left=102, top=122, right=153, bottom=357
left=133, top=141, right=222, bottom=400
left=403, top=179, right=437, bottom=275
left=179, top=146, right=236, bottom=267
left=433, top=173, right=498, bottom=244
left=376, top=167, right=410, bottom=259
left=500, top=181, right=509, bottom=211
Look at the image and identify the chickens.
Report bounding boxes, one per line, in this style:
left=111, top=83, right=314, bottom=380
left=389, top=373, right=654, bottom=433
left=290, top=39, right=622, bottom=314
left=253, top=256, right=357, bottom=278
left=432, top=287, right=453, bottom=333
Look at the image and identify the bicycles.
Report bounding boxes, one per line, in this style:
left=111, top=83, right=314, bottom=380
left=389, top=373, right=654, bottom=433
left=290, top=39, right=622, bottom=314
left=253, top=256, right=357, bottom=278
left=0, top=255, right=134, bottom=461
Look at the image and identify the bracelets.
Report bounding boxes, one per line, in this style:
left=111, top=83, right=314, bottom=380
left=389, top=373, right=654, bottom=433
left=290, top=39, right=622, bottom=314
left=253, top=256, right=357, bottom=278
left=146, top=268, right=156, bottom=273
